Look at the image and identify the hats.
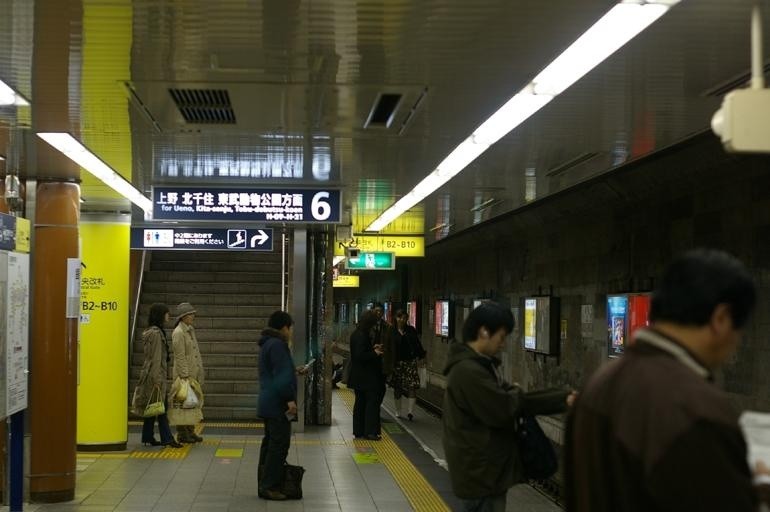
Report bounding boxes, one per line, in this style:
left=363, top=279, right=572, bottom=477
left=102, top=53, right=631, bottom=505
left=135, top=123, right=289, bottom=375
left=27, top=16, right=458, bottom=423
left=175, top=302, right=196, bottom=320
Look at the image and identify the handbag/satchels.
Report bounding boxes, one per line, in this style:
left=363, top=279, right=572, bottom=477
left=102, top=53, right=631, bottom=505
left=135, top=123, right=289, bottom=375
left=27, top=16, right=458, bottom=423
left=280, top=464, right=306, bottom=499
left=341, top=358, right=353, bottom=383
left=167, top=407, right=201, bottom=426
left=513, top=414, right=558, bottom=484
left=142, top=401, right=165, bottom=418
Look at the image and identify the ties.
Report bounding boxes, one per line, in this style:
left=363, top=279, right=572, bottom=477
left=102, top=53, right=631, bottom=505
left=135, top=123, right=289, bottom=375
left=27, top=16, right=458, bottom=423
left=375, top=323, right=379, bottom=331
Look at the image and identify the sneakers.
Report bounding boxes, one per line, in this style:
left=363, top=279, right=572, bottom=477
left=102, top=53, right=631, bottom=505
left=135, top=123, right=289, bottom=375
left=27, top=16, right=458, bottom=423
left=258, top=484, right=287, bottom=500
left=177, top=432, right=203, bottom=443
left=367, top=433, right=381, bottom=440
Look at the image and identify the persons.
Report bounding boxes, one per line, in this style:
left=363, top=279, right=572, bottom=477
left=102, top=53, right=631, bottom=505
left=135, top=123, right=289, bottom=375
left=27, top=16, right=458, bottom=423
left=172, top=301, right=205, bottom=445
left=443, top=298, right=580, bottom=510
left=562, top=247, right=770, bottom=510
left=132, top=306, right=181, bottom=448
left=351, top=308, right=386, bottom=440
left=365, top=303, right=392, bottom=407
left=257, top=310, right=309, bottom=499
left=333, top=340, right=343, bottom=391
left=390, top=309, right=426, bottom=417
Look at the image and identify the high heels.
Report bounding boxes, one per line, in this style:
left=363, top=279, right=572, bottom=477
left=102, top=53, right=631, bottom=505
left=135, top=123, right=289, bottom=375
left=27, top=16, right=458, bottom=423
left=161, top=440, right=183, bottom=448
left=142, top=441, right=160, bottom=447
